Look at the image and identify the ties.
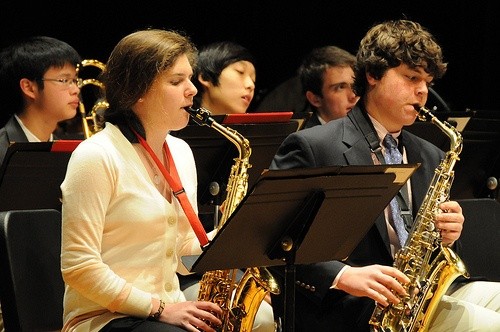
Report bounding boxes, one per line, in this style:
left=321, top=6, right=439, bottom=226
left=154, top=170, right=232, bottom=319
left=382, top=134, right=409, bottom=248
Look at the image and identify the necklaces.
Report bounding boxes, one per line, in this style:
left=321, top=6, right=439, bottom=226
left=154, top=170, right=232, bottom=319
left=150, top=165, right=160, bottom=185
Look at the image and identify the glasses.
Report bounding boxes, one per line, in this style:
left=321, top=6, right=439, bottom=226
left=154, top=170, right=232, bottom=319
left=42, top=78, right=83, bottom=89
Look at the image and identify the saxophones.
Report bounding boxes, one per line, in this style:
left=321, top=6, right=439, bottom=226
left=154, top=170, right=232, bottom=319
left=368, top=102, right=471, bottom=332
left=184, top=106, right=282, bottom=332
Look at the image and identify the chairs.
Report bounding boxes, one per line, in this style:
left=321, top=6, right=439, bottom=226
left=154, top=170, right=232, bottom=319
left=0, top=207, right=64, bottom=332
left=456, top=196, right=500, bottom=280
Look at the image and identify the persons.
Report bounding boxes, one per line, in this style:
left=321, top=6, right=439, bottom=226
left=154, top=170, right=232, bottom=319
left=59, top=30, right=224, bottom=332
left=0, top=36, right=84, bottom=167
left=192, top=37, right=257, bottom=115
left=268, top=22, right=500, bottom=332
left=297, top=46, right=357, bottom=130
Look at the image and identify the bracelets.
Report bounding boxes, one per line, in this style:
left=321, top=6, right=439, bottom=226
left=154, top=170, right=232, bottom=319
left=151, top=300, right=165, bottom=319
